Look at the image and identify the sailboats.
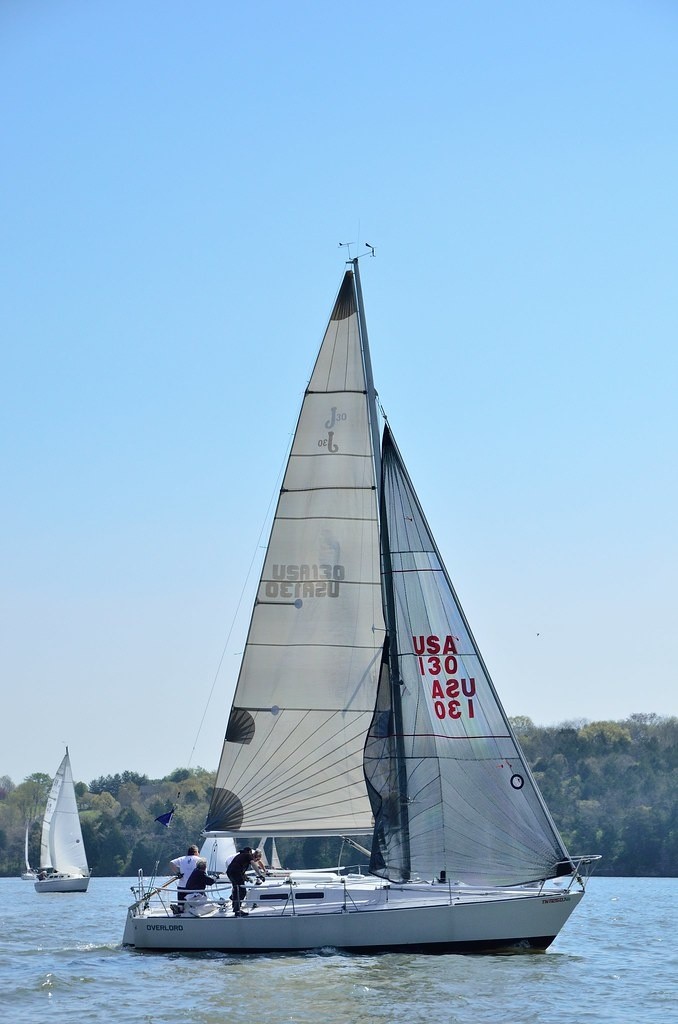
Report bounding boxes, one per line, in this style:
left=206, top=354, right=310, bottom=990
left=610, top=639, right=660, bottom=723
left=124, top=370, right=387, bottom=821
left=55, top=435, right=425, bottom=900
left=21, top=822, right=37, bottom=880
left=35, top=745, right=93, bottom=893
left=124, top=238, right=590, bottom=958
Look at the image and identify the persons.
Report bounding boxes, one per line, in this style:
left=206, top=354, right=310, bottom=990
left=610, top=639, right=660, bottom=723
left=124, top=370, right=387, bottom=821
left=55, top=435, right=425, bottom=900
left=228, top=848, right=273, bottom=916
left=226, top=846, right=251, bottom=868
left=185, top=858, right=220, bottom=918
left=170, top=845, right=207, bottom=913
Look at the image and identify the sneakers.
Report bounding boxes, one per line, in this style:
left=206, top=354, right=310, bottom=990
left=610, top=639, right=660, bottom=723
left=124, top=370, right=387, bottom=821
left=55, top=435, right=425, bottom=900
left=169, top=904, right=180, bottom=914
left=235, top=911, right=248, bottom=916
